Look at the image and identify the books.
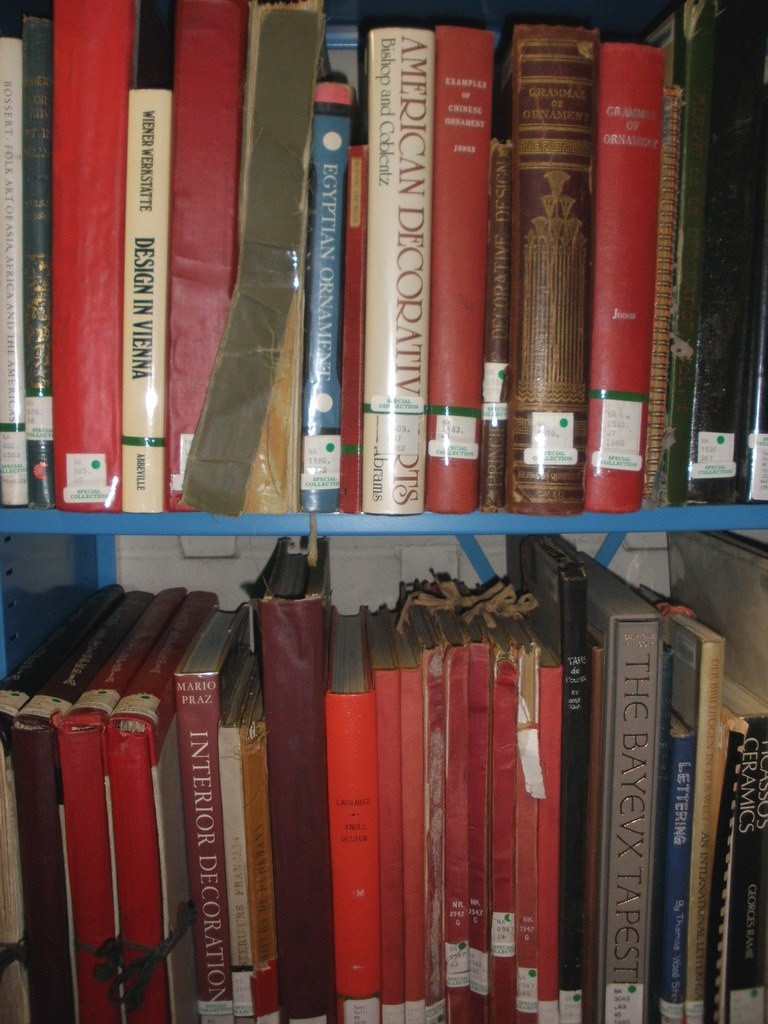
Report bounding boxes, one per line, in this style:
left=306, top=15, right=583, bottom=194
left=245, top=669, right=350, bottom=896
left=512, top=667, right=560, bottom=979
left=0, top=536, right=768, bottom=1024
left=0, top=0, right=768, bottom=512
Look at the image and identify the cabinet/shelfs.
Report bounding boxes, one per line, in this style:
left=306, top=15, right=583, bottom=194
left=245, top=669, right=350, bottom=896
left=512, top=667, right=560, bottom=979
left=0, top=0, right=768, bottom=1024
